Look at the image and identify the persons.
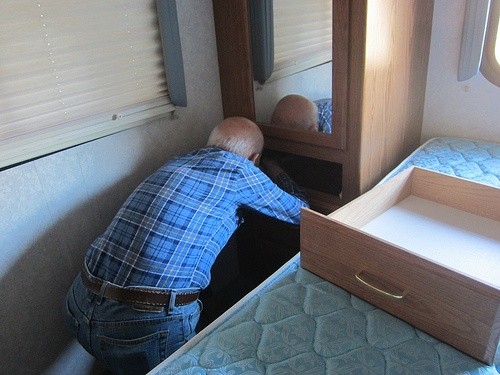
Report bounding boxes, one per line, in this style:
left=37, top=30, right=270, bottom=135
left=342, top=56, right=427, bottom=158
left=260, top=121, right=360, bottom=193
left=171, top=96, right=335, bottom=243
left=271, top=93, right=332, bottom=134
left=63, top=115, right=311, bottom=375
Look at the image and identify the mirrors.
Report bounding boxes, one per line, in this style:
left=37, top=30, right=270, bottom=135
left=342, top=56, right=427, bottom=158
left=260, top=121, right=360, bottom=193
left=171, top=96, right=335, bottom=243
left=246, top=1, right=332, bottom=136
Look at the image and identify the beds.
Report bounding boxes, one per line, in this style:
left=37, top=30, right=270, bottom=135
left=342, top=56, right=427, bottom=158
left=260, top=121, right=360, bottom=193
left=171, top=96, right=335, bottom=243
left=124, top=130, right=499, bottom=375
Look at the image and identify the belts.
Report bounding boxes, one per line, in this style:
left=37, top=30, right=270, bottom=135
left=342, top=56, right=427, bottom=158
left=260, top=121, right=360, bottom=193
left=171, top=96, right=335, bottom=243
left=80, top=257, right=200, bottom=306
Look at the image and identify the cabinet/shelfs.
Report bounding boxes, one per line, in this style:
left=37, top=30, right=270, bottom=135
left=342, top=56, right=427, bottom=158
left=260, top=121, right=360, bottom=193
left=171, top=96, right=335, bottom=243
left=213, top=0, right=437, bottom=271
